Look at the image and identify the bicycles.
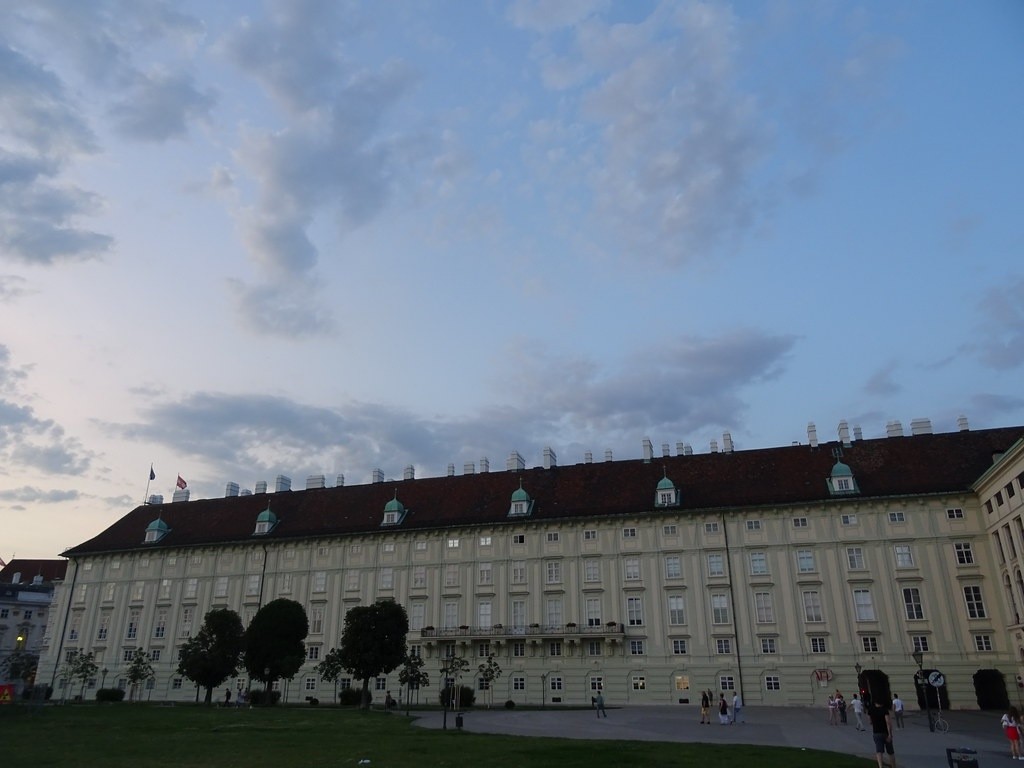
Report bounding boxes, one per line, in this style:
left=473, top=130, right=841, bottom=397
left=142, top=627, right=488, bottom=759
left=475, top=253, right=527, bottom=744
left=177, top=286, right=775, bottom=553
left=934, top=711, right=949, bottom=733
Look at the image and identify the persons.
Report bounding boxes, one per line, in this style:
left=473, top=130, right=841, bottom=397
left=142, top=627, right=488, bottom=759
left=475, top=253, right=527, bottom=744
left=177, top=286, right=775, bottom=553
left=731, top=692, right=745, bottom=723
left=385, top=691, right=392, bottom=713
left=892, top=694, right=904, bottom=730
left=708, top=689, right=733, bottom=725
left=225, top=688, right=231, bottom=704
left=826, top=689, right=847, bottom=727
left=1000, top=706, right=1024, bottom=760
left=1020, top=716, right=1024, bottom=734
left=867, top=698, right=895, bottom=768
left=238, top=687, right=248, bottom=702
left=699, top=691, right=711, bottom=724
left=845, top=694, right=866, bottom=731
left=596, top=691, right=607, bottom=719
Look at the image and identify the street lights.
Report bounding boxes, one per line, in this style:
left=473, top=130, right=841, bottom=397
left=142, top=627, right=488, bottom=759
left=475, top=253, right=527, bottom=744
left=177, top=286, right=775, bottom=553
left=854, top=662, right=866, bottom=713
left=911, top=646, right=935, bottom=732
left=540, top=675, right=546, bottom=708
left=102, top=667, right=109, bottom=689
left=442, top=653, right=453, bottom=730
left=406, top=666, right=412, bottom=716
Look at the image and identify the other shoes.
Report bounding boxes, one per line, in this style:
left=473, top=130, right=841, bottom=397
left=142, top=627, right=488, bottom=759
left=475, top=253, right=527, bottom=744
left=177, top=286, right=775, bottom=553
left=861, top=729, right=865, bottom=731
left=597, top=714, right=607, bottom=718
left=707, top=722, right=710, bottom=725
left=700, top=722, right=704, bottom=724
left=1013, top=756, right=1017, bottom=760
left=856, top=728, right=859, bottom=731
left=1019, top=756, right=1024, bottom=760
